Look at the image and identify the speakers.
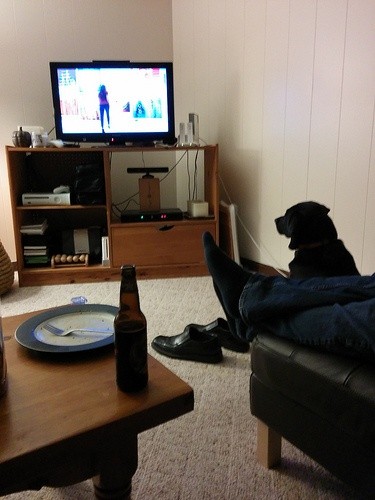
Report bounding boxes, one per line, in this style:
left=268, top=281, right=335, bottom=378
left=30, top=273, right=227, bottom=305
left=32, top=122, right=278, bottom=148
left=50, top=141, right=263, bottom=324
left=189, top=113, right=200, bottom=145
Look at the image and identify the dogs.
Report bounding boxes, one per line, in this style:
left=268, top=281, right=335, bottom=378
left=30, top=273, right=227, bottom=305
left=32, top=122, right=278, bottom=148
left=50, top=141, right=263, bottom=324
left=275, top=201, right=363, bottom=277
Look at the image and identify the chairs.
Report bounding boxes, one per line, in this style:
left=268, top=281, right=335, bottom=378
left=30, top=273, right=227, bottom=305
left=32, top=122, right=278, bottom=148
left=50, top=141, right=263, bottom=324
left=250, top=323, right=375, bottom=500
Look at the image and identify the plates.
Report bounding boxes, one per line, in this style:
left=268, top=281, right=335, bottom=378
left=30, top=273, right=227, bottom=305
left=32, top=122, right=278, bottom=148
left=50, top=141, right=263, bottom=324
left=15, top=304, right=119, bottom=353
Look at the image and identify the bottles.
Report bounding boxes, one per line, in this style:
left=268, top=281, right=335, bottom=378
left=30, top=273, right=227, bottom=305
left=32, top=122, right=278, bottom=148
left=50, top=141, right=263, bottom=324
left=12, top=126, right=31, bottom=148
left=31, top=130, right=48, bottom=148
left=114, top=265, right=148, bottom=394
left=176, top=112, right=200, bottom=147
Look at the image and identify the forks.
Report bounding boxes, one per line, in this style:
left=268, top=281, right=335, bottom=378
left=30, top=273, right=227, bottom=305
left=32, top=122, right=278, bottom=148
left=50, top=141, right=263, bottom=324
left=43, top=322, right=114, bottom=336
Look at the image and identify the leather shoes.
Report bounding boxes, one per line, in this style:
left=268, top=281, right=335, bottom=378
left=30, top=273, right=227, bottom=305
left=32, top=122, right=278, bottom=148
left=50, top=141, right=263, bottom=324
left=184, top=318, right=249, bottom=353
left=152, top=327, right=223, bottom=363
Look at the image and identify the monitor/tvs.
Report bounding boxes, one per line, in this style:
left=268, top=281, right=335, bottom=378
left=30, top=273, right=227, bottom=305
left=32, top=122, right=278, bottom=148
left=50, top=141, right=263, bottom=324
left=49, top=60, right=176, bottom=146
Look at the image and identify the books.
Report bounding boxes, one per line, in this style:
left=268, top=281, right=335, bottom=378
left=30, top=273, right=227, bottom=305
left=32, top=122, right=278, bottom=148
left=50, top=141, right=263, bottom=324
left=20, top=217, right=49, bottom=268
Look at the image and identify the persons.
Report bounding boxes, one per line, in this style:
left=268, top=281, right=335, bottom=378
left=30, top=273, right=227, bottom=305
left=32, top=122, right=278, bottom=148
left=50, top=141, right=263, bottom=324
left=98, top=84, right=110, bottom=134
left=203, top=232, right=375, bottom=373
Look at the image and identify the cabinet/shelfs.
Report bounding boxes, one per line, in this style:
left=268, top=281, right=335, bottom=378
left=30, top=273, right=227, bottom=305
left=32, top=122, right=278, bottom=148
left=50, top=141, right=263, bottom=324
left=5, top=140, right=219, bottom=287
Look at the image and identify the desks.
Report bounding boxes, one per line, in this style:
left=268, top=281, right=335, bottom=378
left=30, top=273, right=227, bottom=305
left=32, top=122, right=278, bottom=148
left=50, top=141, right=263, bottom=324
left=0, top=306, right=196, bottom=500
